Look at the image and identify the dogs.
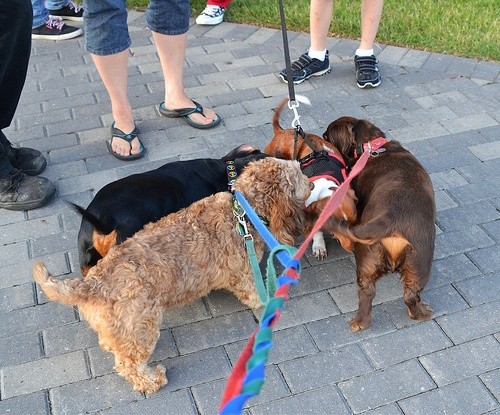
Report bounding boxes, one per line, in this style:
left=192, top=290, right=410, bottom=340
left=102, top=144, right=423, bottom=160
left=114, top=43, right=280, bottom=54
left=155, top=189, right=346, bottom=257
left=260, top=99, right=354, bottom=258
left=30, top=156, right=316, bottom=395
left=321, top=117, right=436, bottom=332
left=76, top=146, right=262, bottom=270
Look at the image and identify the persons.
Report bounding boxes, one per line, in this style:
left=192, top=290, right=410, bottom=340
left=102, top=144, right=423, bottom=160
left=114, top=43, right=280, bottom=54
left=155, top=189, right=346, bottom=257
left=0, top=0, right=56, bottom=211
left=195, top=0, right=232, bottom=25
left=31, top=0, right=84, bottom=40
left=82, top=0, right=222, bottom=161
left=278, top=0, right=384, bottom=89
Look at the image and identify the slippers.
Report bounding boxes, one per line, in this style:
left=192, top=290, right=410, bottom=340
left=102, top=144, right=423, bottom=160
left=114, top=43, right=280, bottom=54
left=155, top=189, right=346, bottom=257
left=159, top=100, right=221, bottom=129
left=106, top=121, right=144, bottom=161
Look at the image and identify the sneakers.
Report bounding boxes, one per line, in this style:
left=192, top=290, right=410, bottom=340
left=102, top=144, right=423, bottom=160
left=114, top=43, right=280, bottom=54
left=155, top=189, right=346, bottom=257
left=48, top=1, right=84, bottom=21
left=354, top=55, right=381, bottom=89
left=195, top=4, right=225, bottom=25
left=0, top=156, right=55, bottom=211
left=31, top=17, right=83, bottom=40
left=278, top=50, right=331, bottom=84
left=0, top=130, right=46, bottom=176
